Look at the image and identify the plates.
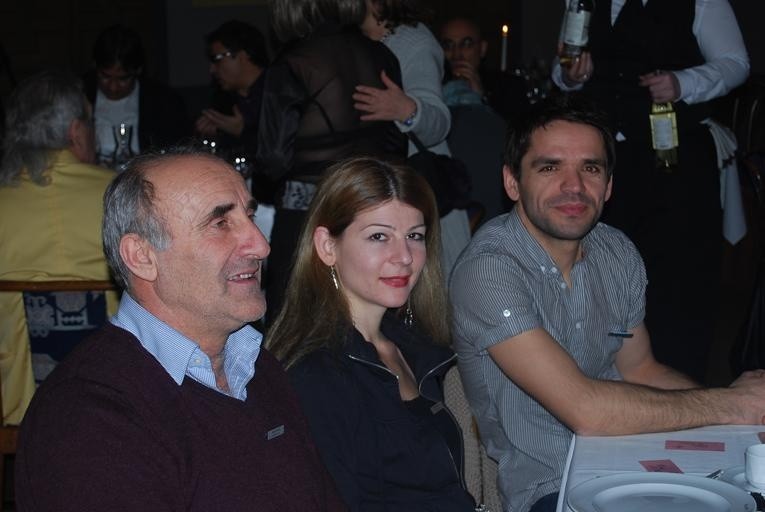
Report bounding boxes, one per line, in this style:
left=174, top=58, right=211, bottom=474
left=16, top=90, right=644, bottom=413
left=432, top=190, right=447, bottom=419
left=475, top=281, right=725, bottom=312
left=718, top=465, right=765, bottom=494
left=567, top=472, right=757, bottom=512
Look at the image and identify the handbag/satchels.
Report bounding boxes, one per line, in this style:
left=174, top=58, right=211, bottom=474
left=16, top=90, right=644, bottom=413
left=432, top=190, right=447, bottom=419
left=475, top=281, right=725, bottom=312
left=405, top=150, right=472, bottom=217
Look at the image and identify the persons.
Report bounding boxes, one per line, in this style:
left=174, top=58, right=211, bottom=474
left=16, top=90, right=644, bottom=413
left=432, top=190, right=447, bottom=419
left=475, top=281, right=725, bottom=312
left=0, top=0, right=765, bottom=512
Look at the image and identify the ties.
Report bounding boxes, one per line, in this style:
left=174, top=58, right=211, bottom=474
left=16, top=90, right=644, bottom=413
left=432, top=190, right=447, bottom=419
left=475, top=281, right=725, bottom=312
left=614, top=1, right=644, bottom=43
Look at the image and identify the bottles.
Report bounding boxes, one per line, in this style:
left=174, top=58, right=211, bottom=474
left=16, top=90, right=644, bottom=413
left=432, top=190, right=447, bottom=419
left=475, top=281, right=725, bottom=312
left=558, top=0, right=591, bottom=64
left=647, top=68, right=681, bottom=170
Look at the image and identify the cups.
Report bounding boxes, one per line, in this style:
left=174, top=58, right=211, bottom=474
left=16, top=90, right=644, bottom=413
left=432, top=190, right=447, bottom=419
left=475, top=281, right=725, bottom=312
left=745, top=443, right=764, bottom=489
left=113, top=123, right=133, bottom=168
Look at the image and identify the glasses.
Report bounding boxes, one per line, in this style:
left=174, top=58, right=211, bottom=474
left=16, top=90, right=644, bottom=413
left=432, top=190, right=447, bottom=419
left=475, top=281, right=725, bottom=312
left=210, top=48, right=240, bottom=63
left=442, top=37, right=480, bottom=50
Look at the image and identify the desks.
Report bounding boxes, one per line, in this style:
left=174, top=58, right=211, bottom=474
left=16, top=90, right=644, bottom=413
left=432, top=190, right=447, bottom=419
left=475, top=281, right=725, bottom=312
left=554, top=425, right=764, bottom=510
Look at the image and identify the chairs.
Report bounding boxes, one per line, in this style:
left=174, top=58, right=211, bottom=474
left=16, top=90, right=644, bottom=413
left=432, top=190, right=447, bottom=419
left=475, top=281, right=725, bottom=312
left=0, top=277, right=124, bottom=453
